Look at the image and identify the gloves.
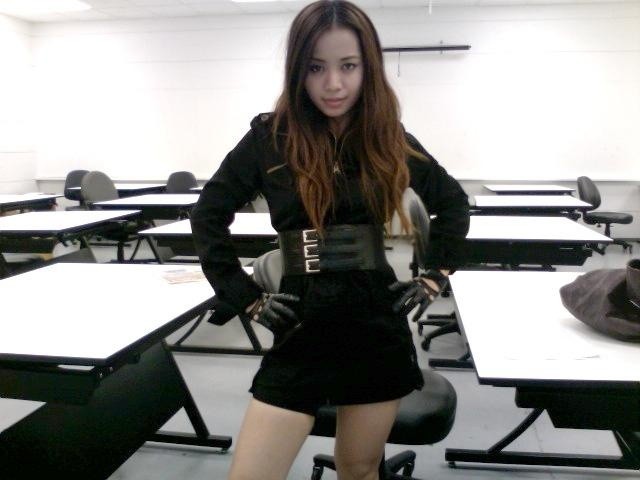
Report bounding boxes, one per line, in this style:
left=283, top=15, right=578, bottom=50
left=247, top=293, right=299, bottom=327
left=388, top=268, right=448, bottom=323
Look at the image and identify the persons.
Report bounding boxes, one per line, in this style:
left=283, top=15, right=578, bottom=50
left=191, top=0, right=469, bottom=480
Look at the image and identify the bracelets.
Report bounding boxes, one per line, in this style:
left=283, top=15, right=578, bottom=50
left=421, top=268, right=449, bottom=290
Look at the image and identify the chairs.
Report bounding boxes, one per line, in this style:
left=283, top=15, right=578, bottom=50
left=401, top=185, right=507, bottom=348
left=65, top=170, right=90, bottom=206
left=247, top=246, right=457, bottom=480
left=166, top=172, right=196, bottom=214
left=577, top=175, right=635, bottom=256
left=81, top=172, right=150, bottom=262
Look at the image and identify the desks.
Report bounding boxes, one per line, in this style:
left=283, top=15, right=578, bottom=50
left=92, top=192, right=202, bottom=266
left=2, top=261, right=256, bottom=478
left=475, top=193, right=593, bottom=271
left=140, top=212, right=276, bottom=357
left=0, top=192, right=69, bottom=216
left=2, top=209, right=140, bottom=264
left=425, top=216, right=615, bottom=370
left=65, top=182, right=167, bottom=246
left=482, top=184, right=577, bottom=194
left=445, top=270, right=637, bottom=471
